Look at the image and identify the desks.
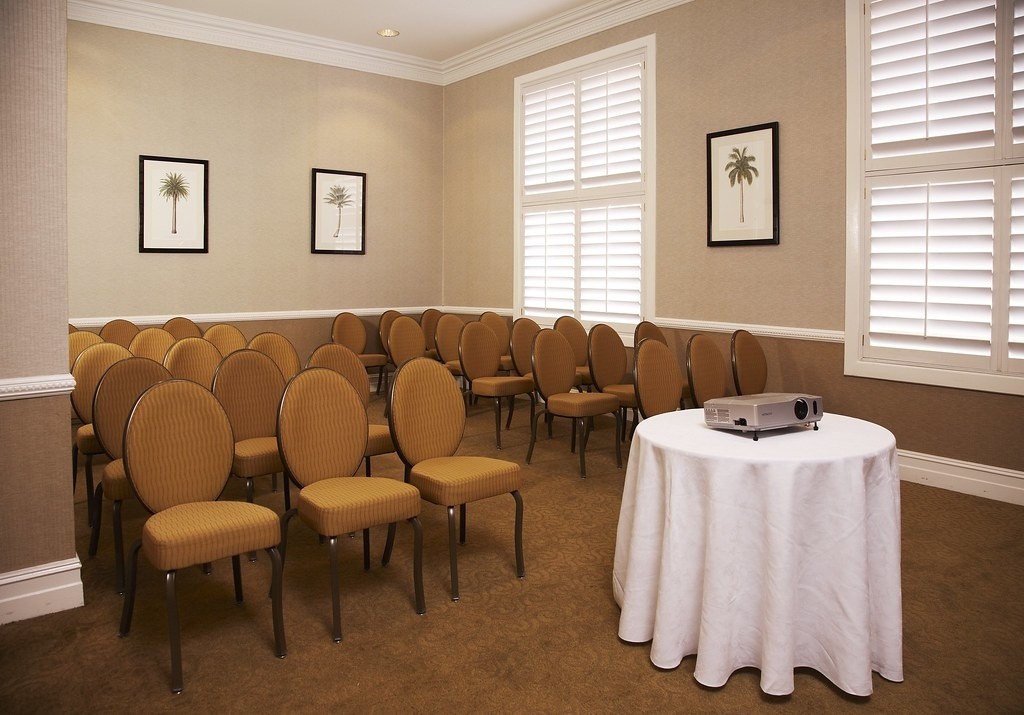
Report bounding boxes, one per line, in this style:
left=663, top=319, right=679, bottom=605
left=608, top=410, right=903, bottom=696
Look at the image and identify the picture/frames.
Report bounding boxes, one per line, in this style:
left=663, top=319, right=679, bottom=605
left=139, top=154, right=209, bottom=255
left=310, top=168, right=367, bottom=253
left=706, top=122, right=779, bottom=245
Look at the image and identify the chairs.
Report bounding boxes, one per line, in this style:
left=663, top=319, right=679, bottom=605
left=68, top=308, right=768, bottom=693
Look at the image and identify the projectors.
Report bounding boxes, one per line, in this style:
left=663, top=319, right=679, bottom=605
left=704, top=393, right=824, bottom=433
